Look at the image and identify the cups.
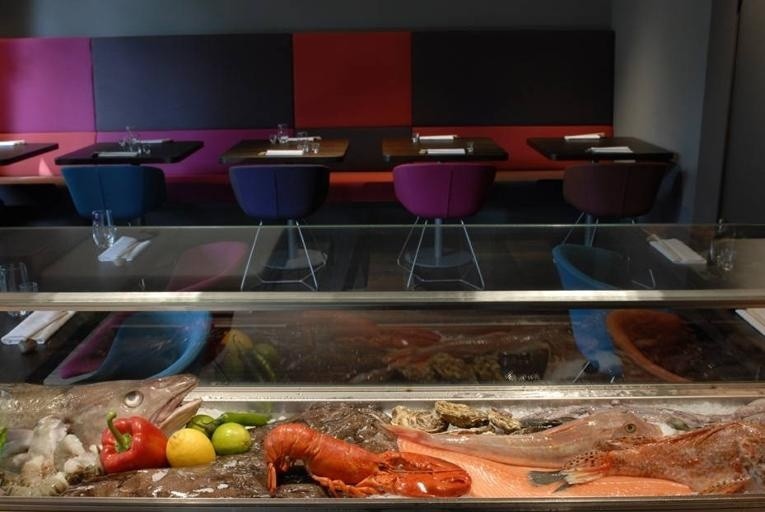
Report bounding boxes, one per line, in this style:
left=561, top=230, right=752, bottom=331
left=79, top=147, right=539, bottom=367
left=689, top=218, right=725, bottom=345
left=117, top=126, right=152, bottom=154
left=0, top=262, right=38, bottom=317
left=91, top=210, right=117, bottom=247
left=269, top=123, right=320, bottom=154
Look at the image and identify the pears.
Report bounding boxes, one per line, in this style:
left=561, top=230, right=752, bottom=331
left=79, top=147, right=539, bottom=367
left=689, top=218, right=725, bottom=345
left=210, top=422, right=251, bottom=455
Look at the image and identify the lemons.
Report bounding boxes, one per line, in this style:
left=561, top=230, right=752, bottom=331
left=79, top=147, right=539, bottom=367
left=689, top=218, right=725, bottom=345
left=221, top=328, right=253, bottom=382
left=166, top=428, right=216, bottom=468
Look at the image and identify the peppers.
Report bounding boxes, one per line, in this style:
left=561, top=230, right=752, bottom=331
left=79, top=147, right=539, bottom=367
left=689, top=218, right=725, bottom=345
left=100, top=412, right=168, bottom=475
left=219, top=412, right=272, bottom=426
left=233, top=337, right=277, bottom=383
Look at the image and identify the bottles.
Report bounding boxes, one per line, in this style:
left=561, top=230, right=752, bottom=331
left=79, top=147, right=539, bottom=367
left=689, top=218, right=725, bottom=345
left=710, top=217, right=737, bottom=272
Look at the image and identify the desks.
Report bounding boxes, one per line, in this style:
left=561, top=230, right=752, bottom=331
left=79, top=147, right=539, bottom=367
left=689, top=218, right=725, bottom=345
left=55, top=142, right=204, bottom=165
left=1, top=142, right=58, bottom=167
left=527, top=137, right=676, bottom=159
left=220, top=140, right=352, bottom=164
left=382, top=138, right=509, bottom=158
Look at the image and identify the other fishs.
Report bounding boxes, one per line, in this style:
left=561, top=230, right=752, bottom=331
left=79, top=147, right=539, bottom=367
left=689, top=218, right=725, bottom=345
left=370, top=404, right=738, bottom=496
left=0, top=371, right=203, bottom=467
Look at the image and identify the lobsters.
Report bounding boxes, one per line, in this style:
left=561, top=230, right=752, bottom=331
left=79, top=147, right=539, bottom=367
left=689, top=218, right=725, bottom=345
left=261, top=420, right=473, bottom=498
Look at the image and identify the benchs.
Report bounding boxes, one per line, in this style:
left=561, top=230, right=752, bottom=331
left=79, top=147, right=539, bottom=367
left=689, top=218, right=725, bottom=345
left=496, top=171, right=563, bottom=201
left=165, top=173, right=229, bottom=200
left=330, top=172, right=392, bottom=202
left=2, top=177, right=61, bottom=203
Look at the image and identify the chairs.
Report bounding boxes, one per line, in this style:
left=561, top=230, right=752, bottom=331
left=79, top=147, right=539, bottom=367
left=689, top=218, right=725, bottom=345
left=62, top=164, right=165, bottom=226
left=553, top=245, right=623, bottom=384
left=393, top=162, right=496, bottom=289
left=165, top=241, right=246, bottom=290
left=563, top=162, right=666, bottom=244
left=229, top=164, right=330, bottom=290
